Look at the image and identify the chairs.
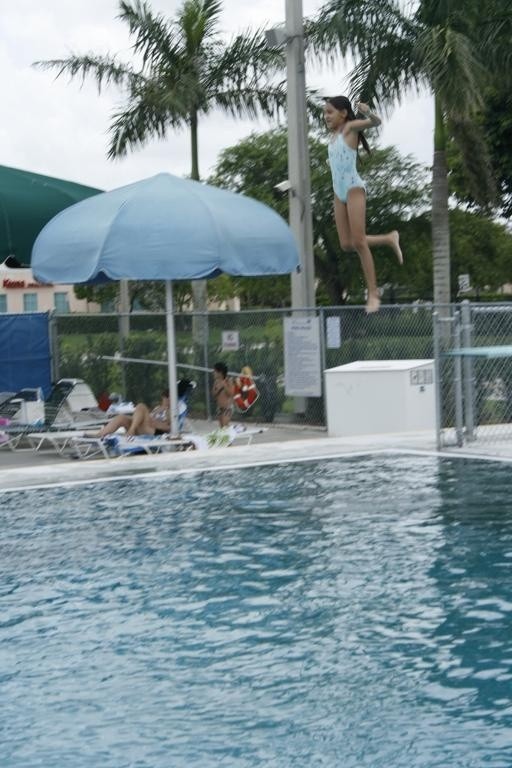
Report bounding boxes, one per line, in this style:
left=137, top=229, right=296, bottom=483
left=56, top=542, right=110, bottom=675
left=72, top=380, right=197, bottom=459
left=0, top=376, right=85, bottom=451
left=0, top=387, right=40, bottom=419
left=66, top=379, right=134, bottom=429
left=28, top=426, right=128, bottom=456
left=117, top=426, right=269, bottom=453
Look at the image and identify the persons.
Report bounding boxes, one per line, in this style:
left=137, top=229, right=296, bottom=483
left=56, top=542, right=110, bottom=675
left=324, top=96, right=403, bottom=314
left=84, top=362, right=258, bottom=439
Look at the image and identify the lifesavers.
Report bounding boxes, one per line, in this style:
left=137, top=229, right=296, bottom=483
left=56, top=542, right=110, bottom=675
left=233, top=376, right=258, bottom=410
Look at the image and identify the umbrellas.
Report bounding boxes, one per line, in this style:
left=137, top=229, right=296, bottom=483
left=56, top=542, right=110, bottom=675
left=31, top=172, right=303, bottom=439
